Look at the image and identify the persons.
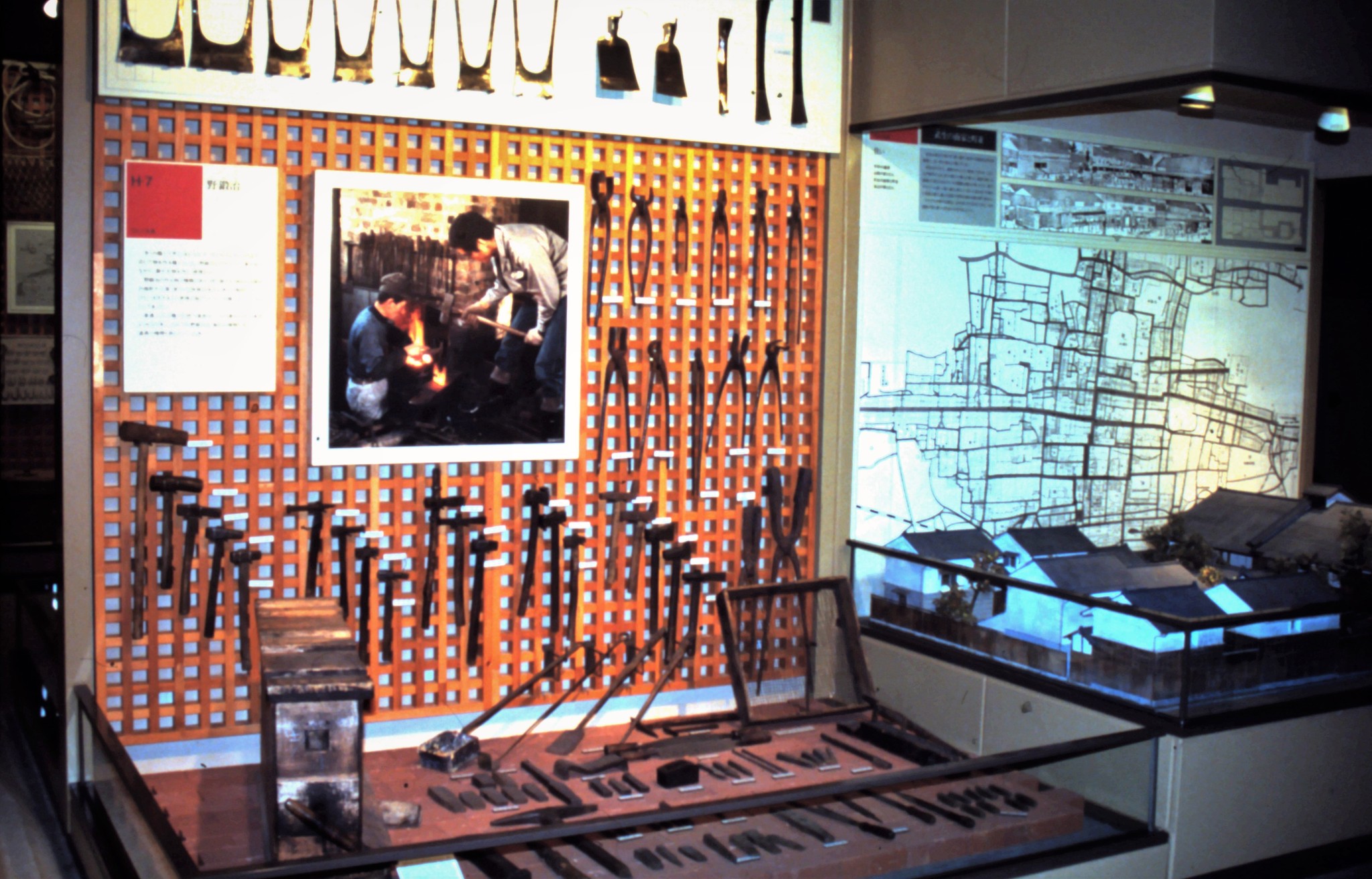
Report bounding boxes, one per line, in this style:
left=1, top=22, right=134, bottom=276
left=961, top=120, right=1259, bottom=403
left=448, top=210, right=568, bottom=444
left=346, top=270, right=439, bottom=430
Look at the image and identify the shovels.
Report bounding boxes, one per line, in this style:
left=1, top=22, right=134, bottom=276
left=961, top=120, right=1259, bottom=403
left=477, top=630, right=630, bottom=771
left=655, top=18, right=688, bottom=98
left=597, top=10, right=642, bottom=92
left=545, top=625, right=669, bottom=755
left=418, top=643, right=580, bottom=775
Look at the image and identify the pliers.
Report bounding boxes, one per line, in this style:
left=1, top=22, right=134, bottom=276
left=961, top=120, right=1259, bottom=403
left=589, top=167, right=806, bottom=501
left=766, top=466, right=817, bottom=695
left=734, top=505, right=762, bottom=675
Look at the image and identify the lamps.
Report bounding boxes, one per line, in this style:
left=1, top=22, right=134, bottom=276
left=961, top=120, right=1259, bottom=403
left=1312, top=106, right=1351, bottom=145
left=1177, top=81, right=1216, bottom=119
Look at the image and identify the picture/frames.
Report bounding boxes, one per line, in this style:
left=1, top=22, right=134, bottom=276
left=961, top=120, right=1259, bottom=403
left=0, top=213, right=60, bottom=324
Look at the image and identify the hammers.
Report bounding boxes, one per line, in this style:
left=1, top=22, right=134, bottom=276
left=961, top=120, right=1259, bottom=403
left=630, top=716, right=723, bottom=739
left=603, top=646, right=688, bottom=755
left=438, top=292, right=528, bottom=338
left=113, top=418, right=729, bottom=679
left=662, top=722, right=719, bottom=739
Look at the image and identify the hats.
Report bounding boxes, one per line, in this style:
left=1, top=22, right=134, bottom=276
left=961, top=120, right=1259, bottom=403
left=381, top=272, right=422, bottom=301
